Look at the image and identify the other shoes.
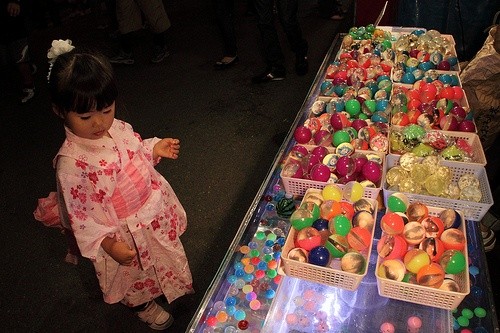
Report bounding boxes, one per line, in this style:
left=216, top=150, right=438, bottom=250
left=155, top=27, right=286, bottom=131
left=148, top=52, right=171, bottom=64
left=294, top=55, right=310, bottom=76
left=135, top=301, right=174, bottom=330
left=212, top=56, right=240, bottom=69
left=16, top=87, right=40, bottom=105
left=316, top=6, right=350, bottom=21
left=115, top=54, right=137, bottom=65
left=251, top=65, right=289, bottom=83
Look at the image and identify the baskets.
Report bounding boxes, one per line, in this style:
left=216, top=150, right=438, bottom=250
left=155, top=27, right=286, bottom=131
left=279, top=188, right=379, bottom=292
left=375, top=205, right=470, bottom=311
left=277, top=26, right=494, bottom=221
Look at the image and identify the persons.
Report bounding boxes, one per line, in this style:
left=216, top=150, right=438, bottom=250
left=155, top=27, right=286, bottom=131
left=32, top=38, right=194, bottom=330
left=458, top=131, right=500, bottom=253
left=395, top=0, right=491, bottom=62
left=250, top=0, right=309, bottom=83
left=215, top=0, right=241, bottom=68
left=0, top=0, right=37, bottom=103
left=331, top=0, right=352, bottom=20
left=106, top=0, right=172, bottom=65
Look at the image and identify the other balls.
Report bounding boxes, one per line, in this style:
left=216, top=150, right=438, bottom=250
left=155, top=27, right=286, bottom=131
left=276, top=24, right=493, bottom=294
left=379, top=306, right=486, bottom=333
left=202, top=195, right=286, bottom=333
